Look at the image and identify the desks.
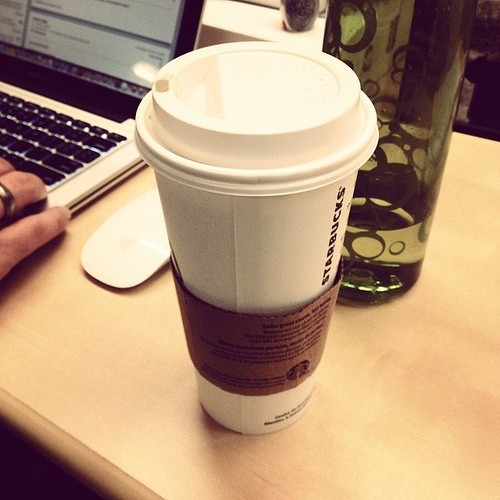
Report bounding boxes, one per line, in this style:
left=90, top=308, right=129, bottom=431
left=0, top=1, right=500, bottom=500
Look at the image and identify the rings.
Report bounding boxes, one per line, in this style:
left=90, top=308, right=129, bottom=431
left=0, top=182, right=16, bottom=218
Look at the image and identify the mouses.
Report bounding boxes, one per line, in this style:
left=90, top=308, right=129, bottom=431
left=80, top=186, right=171, bottom=290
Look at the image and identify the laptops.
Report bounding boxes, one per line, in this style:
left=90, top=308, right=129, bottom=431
left=0, top=0, right=205, bottom=225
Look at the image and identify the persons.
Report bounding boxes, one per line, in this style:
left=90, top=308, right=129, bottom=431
left=0, top=157, right=72, bottom=284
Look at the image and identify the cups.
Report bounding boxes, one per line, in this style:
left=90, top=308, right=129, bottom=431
left=133, top=41, right=379, bottom=437
left=278, top=0, right=319, bottom=33
left=321, top=0, right=479, bottom=305
